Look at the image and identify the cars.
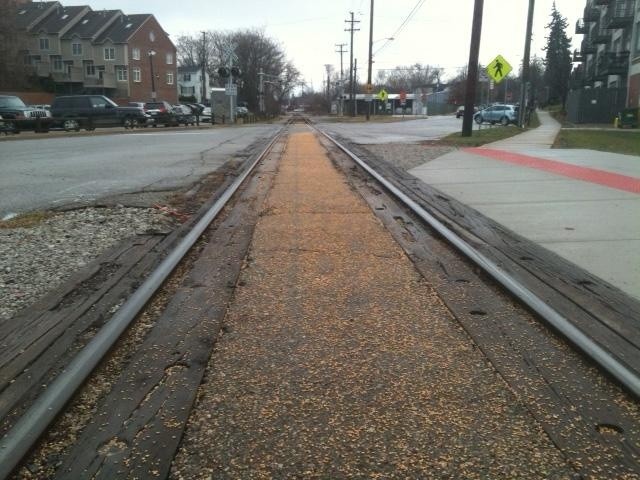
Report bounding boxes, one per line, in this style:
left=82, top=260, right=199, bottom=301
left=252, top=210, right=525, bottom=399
left=0, top=94, right=249, bottom=134
left=474, top=104, right=519, bottom=126
left=456, top=105, right=465, bottom=117
